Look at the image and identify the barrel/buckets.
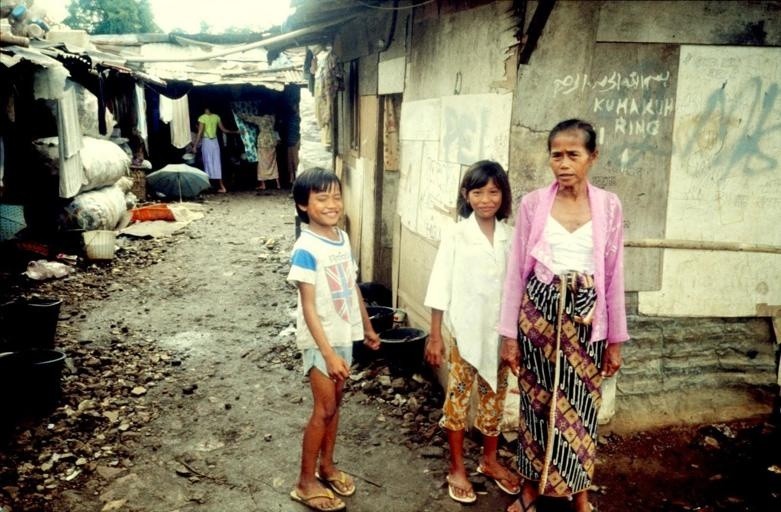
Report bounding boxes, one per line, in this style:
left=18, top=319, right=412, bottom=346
left=30, top=349, right=67, bottom=422
left=378, top=323, right=429, bottom=379
left=81, top=228, right=119, bottom=262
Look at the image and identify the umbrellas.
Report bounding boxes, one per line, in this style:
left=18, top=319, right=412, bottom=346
left=146, top=163, right=213, bottom=204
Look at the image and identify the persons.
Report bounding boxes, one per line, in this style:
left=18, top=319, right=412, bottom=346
left=494, top=116, right=631, bottom=512
left=192, top=101, right=246, bottom=194
left=284, top=165, right=384, bottom=512
left=287, top=102, right=301, bottom=197
left=426, top=158, right=521, bottom=504
left=235, top=102, right=283, bottom=192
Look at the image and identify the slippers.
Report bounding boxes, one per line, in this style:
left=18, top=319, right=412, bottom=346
left=476, top=467, right=521, bottom=495
left=290, top=488, right=346, bottom=511
left=519, top=496, right=536, bottom=512
left=315, top=470, right=356, bottom=496
left=446, top=475, right=477, bottom=503
